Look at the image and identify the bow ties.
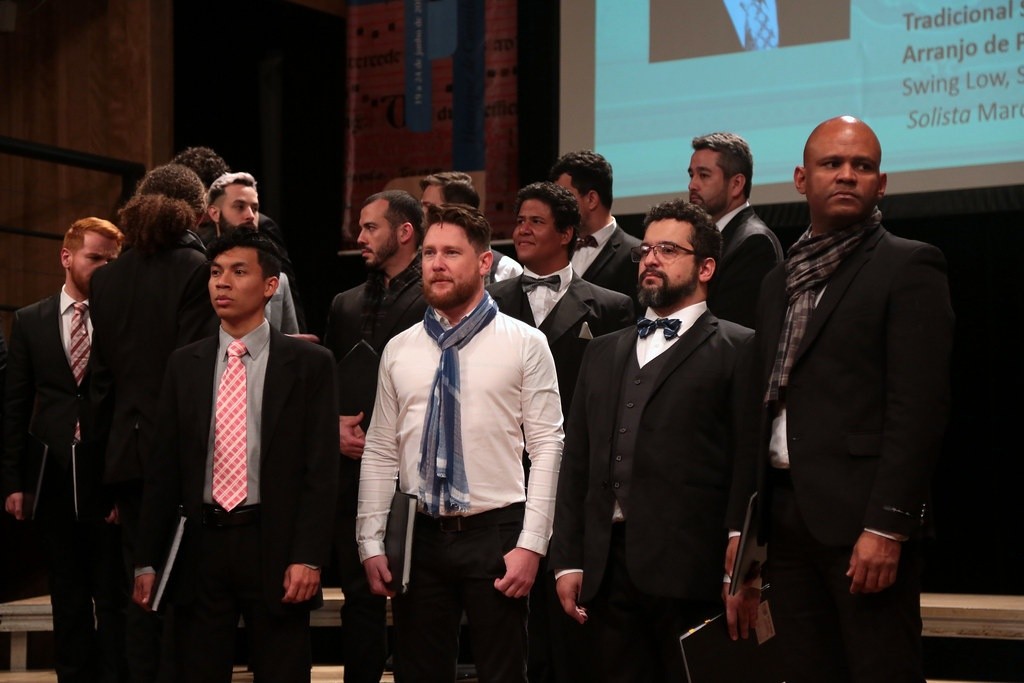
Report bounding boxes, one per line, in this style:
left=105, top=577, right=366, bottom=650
left=635, top=316, right=681, bottom=340
left=521, top=274, right=561, bottom=292
left=574, top=235, right=598, bottom=251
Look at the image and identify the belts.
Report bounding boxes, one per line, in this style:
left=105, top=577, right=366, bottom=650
left=612, top=525, right=624, bottom=535
left=765, top=471, right=792, bottom=489
left=201, top=505, right=258, bottom=527
left=416, top=509, right=522, bottom=532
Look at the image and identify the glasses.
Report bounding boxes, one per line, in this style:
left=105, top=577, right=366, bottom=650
left=630, top=242, right=696, bottom=264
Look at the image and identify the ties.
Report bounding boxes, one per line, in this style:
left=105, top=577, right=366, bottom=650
left=211, top=339, right=247, bottom=511
left=71, top=303, right=91, bottom=442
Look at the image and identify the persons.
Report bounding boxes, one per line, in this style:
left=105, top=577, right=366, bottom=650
left=723, top=114, right=951, bottom=683
left=1, top=130, right=785, bottom=683
